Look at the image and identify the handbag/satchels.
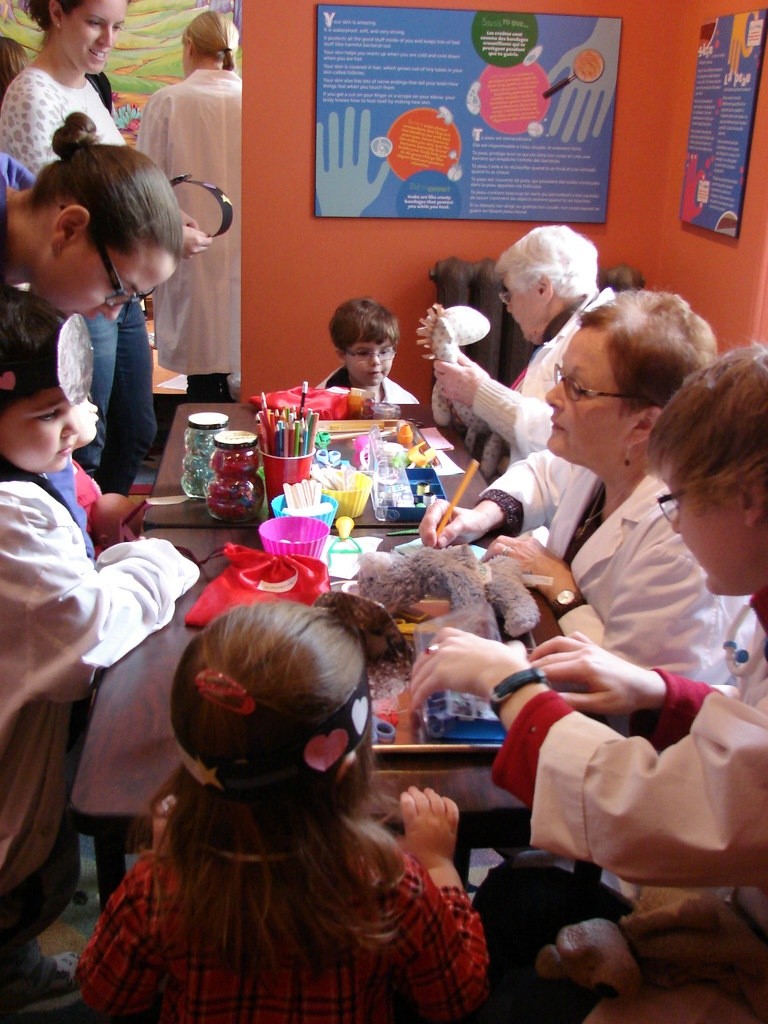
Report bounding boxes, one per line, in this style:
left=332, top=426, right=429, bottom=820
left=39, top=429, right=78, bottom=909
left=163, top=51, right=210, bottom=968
left=184, top=542, right=331, bottom=626
left=250, top=384, right=350, bottom=421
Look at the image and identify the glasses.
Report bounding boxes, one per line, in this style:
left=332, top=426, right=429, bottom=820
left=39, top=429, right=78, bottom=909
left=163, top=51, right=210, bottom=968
left=658, top=486, right=701, bottom=522
left=60, top=205, right=157, bottom=308
left=498, top=289, right=518, bottom=306
left=553, top=363, right=659, bottom=408
left=343, top=346, right=397, bottom=362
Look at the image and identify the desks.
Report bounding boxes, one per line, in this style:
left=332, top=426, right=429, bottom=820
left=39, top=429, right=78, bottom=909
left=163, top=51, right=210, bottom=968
left=142, top=403, right=489, bottom=528
left=66, top=524, right=567, bottom=913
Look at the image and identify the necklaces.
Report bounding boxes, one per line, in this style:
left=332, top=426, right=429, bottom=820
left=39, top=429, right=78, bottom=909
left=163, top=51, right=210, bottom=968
left=578, top=484, right=627, bottom=540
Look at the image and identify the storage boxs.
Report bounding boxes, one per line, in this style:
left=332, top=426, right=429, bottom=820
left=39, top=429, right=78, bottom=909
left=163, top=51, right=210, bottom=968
left=377, top=468, right=447, bottom=522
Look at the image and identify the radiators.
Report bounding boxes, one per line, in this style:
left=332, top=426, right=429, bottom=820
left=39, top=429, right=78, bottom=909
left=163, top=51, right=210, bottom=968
left=426, top=255, right=646, bottom=457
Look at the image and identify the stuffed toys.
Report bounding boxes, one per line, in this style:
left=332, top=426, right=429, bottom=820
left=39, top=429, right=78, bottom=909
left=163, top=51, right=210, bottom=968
left=537, top=887, right=767, bottom=1024
left=415, top=304, right=505, bottom=477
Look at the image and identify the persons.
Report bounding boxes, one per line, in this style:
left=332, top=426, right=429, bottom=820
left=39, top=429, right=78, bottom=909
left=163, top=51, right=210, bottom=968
left=433, top=224, right=616, bottom=467
left=418, top=288, right=756, bottom=685
left=408, top=343, right=768, bottom=1024
left=0, top=0, right=212, bottom=552
left=0, top=111, right=183, bottom=563
left=134, top=10, right=242, bottom=403
left=77, top=601, right=490, bottom=1023
left=0, top=283, right=202, bottom=1009
left=315, top=300, right=421, bottom=418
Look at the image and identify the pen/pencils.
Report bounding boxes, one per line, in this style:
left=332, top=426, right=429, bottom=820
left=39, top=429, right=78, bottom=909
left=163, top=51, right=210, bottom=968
left=436, top=458, right=480, bottom=538
left=255, top=381, right=319, bottom=458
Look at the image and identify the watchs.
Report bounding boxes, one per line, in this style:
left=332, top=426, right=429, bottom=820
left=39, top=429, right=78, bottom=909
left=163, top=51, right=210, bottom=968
left=552, top=590, right=583, bottom=613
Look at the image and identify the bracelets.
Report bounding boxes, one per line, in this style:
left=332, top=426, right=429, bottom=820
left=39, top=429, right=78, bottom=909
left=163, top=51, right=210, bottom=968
left=490, top=668, right=547, bottom=717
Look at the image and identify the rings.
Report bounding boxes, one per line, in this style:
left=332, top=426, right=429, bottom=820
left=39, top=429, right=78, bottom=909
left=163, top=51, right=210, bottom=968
left=425, top=645, right=438, bottom=655
left=503, top=546, right=511, bottom=556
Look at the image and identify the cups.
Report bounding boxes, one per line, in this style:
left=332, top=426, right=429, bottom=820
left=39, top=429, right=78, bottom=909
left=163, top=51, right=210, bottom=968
left=258, top=447, right=315, bottom=515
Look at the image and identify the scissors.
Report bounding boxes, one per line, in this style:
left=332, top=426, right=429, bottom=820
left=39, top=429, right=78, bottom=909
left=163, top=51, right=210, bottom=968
left=316, top=449, right=341, bottom=469
left=315, top=431, right=330, bottom=451
left=371, top=716, right=396, bottom=744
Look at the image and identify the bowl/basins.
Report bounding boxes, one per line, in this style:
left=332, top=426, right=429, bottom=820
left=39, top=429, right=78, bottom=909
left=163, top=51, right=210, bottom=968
left=260, top=517, right=330, bottom=561
left=318, top=471, right=373, bottom=518
left=271, top=492, right=338, bottom=528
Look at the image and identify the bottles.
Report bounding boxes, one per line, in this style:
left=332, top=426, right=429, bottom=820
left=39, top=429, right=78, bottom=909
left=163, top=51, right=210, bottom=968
left=204, top=432, right=265, bottom=521
left=180, top=413, right=230, bottom=498
